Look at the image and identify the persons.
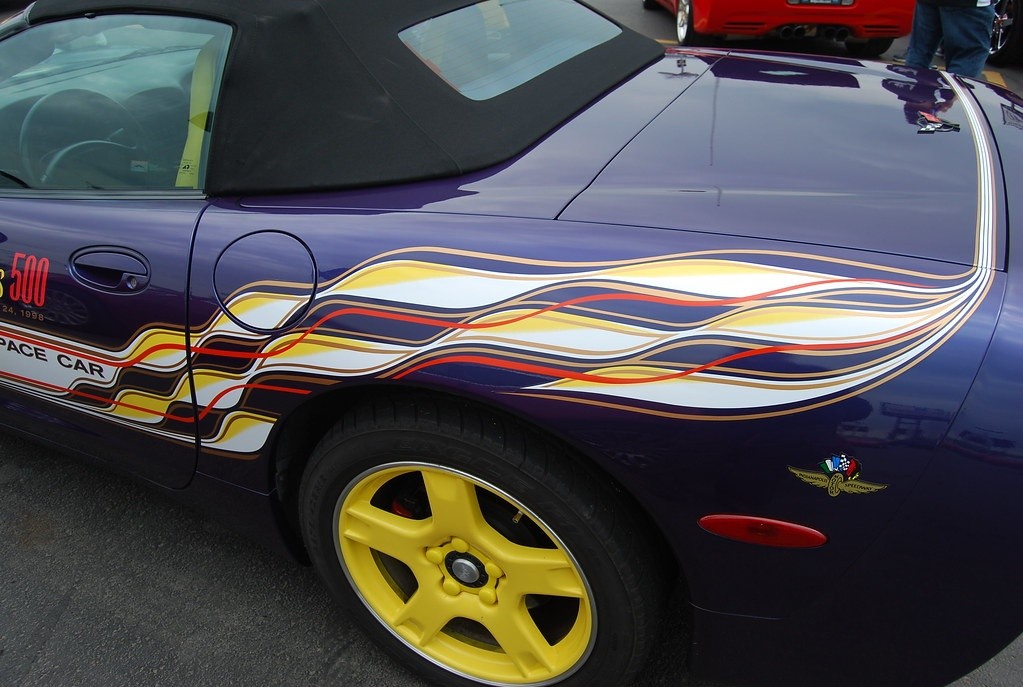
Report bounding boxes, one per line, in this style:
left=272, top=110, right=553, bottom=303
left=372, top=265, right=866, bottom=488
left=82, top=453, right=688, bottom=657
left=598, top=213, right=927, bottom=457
left=882, top=64, right=959, bottom=125
left=970, top=79, right=1023, bottom=108
left=906, top=0, right=994, bottom=78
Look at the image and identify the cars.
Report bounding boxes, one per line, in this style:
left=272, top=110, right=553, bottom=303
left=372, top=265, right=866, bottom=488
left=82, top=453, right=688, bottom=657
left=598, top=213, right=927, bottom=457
left=640, top=0, right=917, bottom=60
left=0, top=0, right=1023, bottom=687
